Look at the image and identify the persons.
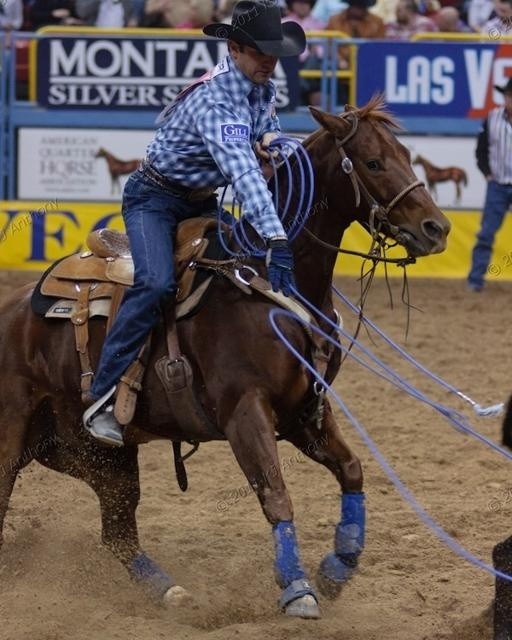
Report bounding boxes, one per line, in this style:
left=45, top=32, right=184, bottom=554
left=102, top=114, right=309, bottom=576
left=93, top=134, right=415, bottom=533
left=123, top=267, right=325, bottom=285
left=467, top=78, right=512, bottom=290
left=81, top=0, right=298, bottom=449
left=0, top=0, right=512, bottom=110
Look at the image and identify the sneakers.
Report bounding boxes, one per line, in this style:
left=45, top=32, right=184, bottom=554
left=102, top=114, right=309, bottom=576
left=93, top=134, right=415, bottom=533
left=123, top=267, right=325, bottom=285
left=85, top=405, right=126, bottom=447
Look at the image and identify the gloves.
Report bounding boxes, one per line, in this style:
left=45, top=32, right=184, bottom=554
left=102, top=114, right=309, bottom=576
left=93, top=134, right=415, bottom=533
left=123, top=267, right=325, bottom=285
left=268, top=239, right=298, bottom=297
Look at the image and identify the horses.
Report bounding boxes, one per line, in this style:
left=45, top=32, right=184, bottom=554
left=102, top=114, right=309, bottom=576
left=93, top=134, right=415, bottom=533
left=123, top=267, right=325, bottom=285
left=94, top=148, right=143, bottom=194
left=413, top=154, right=467, bottom=203
left=1, top=91, right=454, bottom=621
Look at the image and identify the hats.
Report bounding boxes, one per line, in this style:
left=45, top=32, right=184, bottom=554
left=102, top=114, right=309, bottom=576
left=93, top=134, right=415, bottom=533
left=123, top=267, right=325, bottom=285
left=203, top=2, right=306, bottom=57
